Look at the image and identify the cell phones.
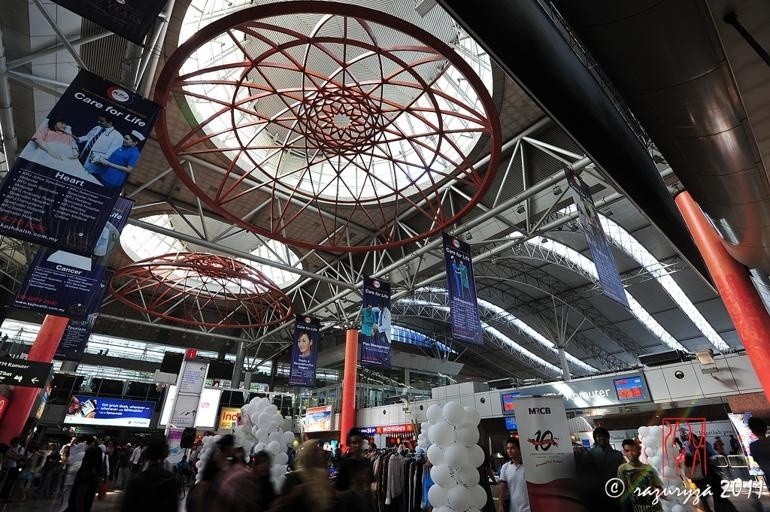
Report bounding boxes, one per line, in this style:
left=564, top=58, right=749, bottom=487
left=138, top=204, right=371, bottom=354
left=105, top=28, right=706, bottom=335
left=71, top=400, right=97, bottom=417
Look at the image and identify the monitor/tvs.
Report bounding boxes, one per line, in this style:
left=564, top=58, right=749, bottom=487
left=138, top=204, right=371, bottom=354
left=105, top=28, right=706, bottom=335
left=611, top=372, right=653, bottom=403
left=500, top=390, right=522, bottom=415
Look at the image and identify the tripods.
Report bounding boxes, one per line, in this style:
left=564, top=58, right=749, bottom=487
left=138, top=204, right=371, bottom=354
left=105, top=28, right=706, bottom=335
left=176, top=449, right=186, bottom=497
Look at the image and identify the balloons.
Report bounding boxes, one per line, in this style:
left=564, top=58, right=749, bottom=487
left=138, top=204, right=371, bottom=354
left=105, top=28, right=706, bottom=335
left=233, top=397, right=295, bottom=494
left=415, top=401, right=486, bottom=511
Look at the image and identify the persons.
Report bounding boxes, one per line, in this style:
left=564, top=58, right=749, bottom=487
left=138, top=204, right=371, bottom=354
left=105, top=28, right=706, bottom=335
left=297, top=331, right=313, bottom=360
left=0, top=424, right=770, bottom=512
left=30, top=113, right=145, bottom=189
left=360, top=300, right=392, bottom=344
left=36, top=382, right=53, bottom=419
left=451, top=255, right=471, bottom=301
left=576, top=201, right=609, bottom=257
left=67, top=396, right=98, bottom=419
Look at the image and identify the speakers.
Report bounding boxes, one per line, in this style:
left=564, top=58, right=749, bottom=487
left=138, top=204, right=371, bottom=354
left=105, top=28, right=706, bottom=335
left=180, top=427, right=197, bottom=448
